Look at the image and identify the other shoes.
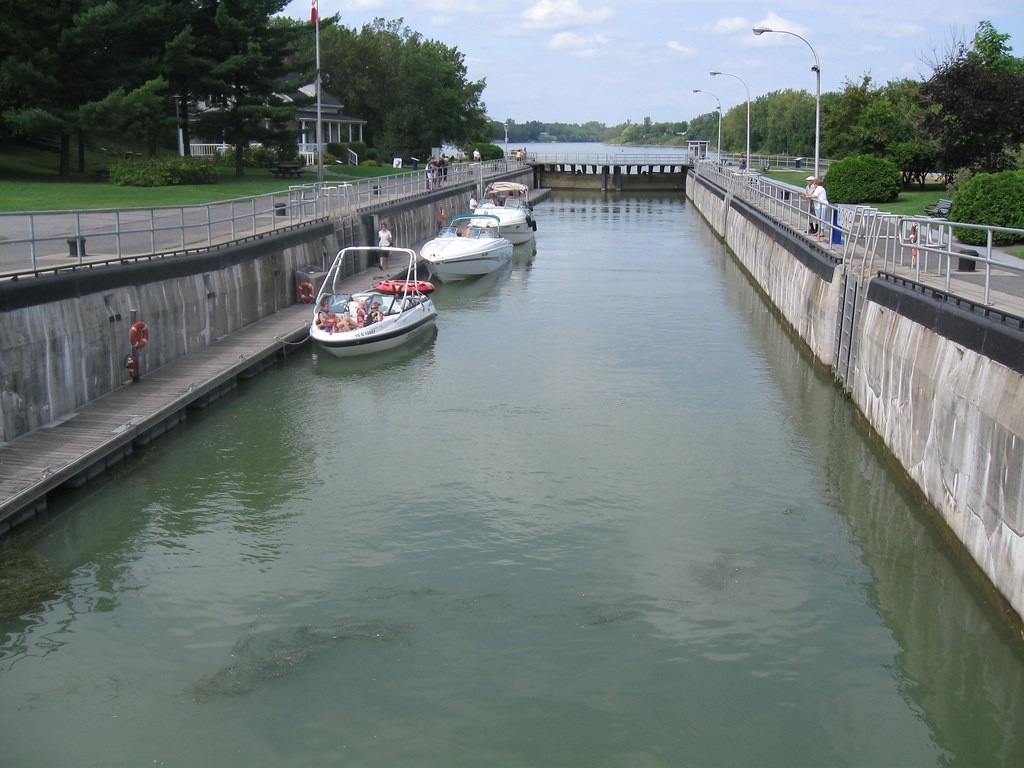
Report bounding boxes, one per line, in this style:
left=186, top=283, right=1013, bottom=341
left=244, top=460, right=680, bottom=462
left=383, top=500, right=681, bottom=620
left=379, top=266, right=383, bottom=270
left=805, top=229, right=815, bottom=233
left=810, top=229, right=817, bottom=234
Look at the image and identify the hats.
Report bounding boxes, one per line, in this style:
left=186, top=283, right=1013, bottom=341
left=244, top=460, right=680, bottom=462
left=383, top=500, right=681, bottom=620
left=805, top=176, right=814, bottom=181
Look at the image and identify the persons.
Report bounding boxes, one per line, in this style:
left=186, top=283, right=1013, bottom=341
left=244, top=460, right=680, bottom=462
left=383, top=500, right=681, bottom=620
left=507, top=191, right=516, bottom=199
left=456, top=148, right=465, bottom=163
left=738, top=156, right=747, bottom=171
left=425, top=153, right=448, bottom=190
left=798, top=176, right=829, bottom=237
left=473, top=148, right=480, bottom=161
left=470, top=193, right=477, bottom=214
left=319, top=299, right=383, bottom=333
left=378, top=223, right=392, bottom=269
left=516, top=147, right=527, bottom=168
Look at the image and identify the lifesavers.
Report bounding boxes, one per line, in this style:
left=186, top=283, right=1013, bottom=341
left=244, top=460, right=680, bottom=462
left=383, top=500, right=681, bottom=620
left=297, top=283, right=314, bottom=302
left=130, top=321, right=148, bottom=348
left=910, top=223, right=918, bottom=244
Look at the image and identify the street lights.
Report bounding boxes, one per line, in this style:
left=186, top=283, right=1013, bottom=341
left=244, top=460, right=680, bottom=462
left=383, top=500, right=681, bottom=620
left=752, top=25, right=821, bottom=179
left=504, top=124, right=509, bottom=156
left=710, top=71, right=749, bottom=174
left=693, top=90, right=721, bottom=164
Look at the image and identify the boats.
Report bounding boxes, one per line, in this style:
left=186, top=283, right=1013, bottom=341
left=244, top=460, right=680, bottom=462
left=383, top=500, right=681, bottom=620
left=469, top=182, right=537, bottom=245
left=309, top=246, right=439, bottom=356
left=419, top=211, right=514, bottom=283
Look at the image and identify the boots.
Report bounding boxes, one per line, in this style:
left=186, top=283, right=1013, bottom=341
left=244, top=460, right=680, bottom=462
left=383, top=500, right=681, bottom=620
left=820, top=229, right=824, bottom=237
left=811, top=232, right=818, bottom=236
